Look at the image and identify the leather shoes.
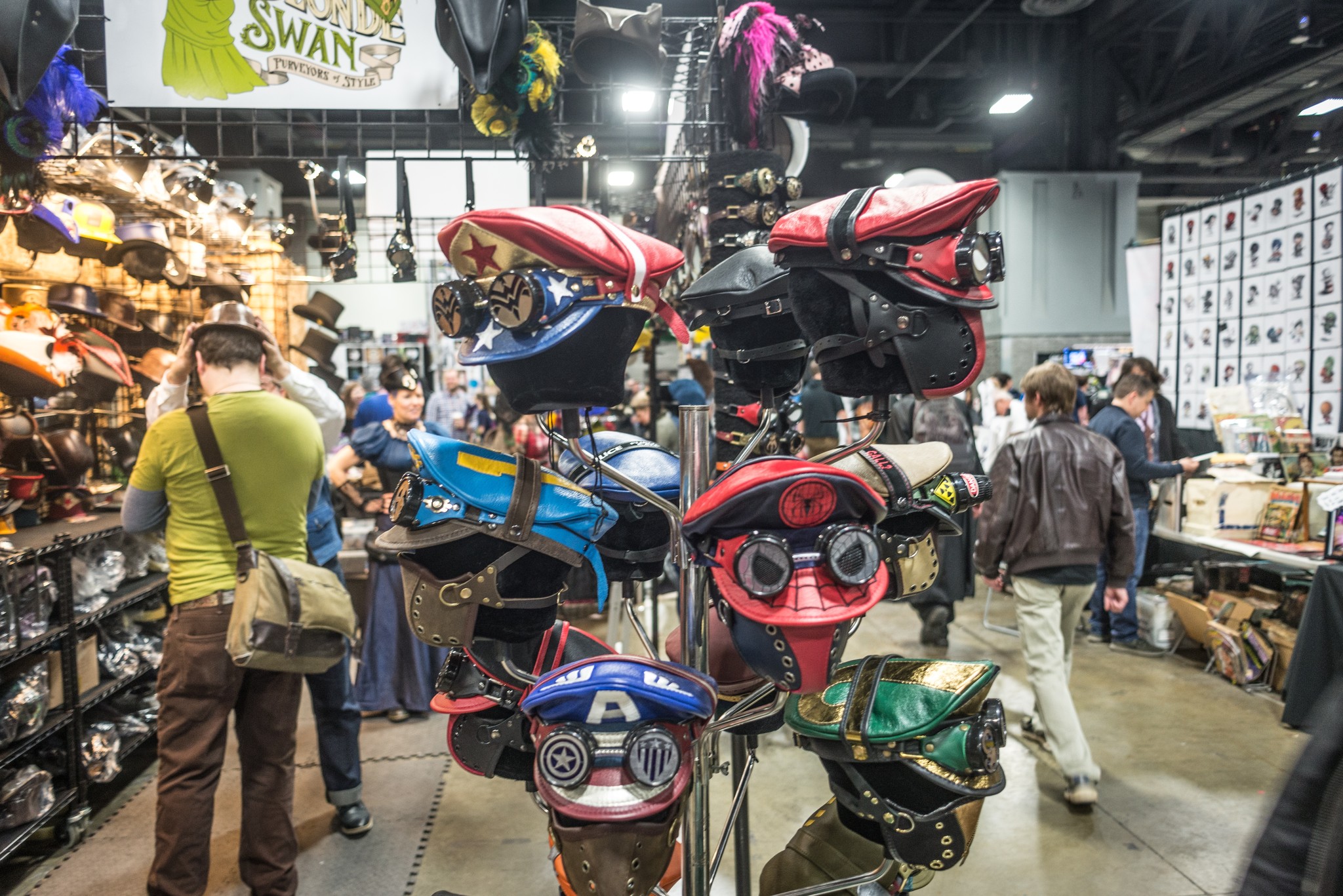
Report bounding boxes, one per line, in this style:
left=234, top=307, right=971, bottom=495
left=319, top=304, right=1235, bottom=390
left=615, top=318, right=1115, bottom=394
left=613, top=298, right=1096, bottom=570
left=335, top=802, right=372, bottom=834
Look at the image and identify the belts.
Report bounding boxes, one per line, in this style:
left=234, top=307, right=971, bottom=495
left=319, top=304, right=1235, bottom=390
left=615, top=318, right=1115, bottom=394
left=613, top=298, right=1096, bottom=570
left=179, top=590, right=235, bottom=610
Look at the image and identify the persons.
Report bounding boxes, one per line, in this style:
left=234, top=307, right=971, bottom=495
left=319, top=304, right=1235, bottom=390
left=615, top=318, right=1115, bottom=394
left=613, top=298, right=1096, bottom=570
left=1330, top=445, right=1343, bottom=466
left=424, top=370, right=552, bottom=468
left=145, top=313, right=372, bottom=833
left=614, top=358, right=717, bottom=473
left=329, top=356, right=452, bottom=718
left=973, top=360, right=1135, bottom=804
left=121, top=302, right=326, bottom=896
left=1297, top=452, right=1316, bottom=478
left=1071, top=357, right=1199, bottom=655
left=801, top=359, right=1012, bottom=646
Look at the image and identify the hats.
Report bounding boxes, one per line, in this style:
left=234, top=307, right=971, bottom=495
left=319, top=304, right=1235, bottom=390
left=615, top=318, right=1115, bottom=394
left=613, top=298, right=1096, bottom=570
left=677, top=459, right=894, bottom=627
left=438, top=210, right=690, bottom=364
left=290, top=327, right=341, bottom=373
left=375, top=433, right=619, bottom=612
left=556, top=428, right=681, bottom=563
left=681, top=248, right=797, bottom=323
left=519, top=656, right=720, bottom=824
left=293, top=292, right=343, bottom=334
left=785, top=658, right=1008, bottom=797
left=0, top=191, right=206, bottom=483
left=429, top=620, right=622, bottom=777
left=190, top=301, right=267, bottom=340
left=765, top=179, right=1002, bottom=310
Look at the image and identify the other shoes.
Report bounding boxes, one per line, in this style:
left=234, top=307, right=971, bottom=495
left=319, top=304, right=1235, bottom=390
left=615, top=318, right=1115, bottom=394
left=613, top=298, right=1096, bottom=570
left=1087, top=628, right=1111, bottom=642
left=1064, top=777, right=1098, bottom=805
left=1110, top=631, right=1165, bottom=657
left=389, top=706, right=410, bottom=722
left=1021, top=717, right=1051, bottom=753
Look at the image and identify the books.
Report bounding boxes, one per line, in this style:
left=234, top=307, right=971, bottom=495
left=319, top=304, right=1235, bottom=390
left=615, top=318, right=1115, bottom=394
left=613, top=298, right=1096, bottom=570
left=1206, top=621, right=1272, bottom=685
left=1217, top=411, right=1329, bottom=542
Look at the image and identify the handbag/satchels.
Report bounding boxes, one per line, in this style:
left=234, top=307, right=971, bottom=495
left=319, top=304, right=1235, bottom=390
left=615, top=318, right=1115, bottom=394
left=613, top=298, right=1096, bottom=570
left=224, top=549, right=357, bottom=675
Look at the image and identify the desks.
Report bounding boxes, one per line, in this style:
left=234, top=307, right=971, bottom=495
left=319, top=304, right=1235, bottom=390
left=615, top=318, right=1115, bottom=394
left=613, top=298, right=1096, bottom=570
left=1150, top=524, right=1339, bottom=574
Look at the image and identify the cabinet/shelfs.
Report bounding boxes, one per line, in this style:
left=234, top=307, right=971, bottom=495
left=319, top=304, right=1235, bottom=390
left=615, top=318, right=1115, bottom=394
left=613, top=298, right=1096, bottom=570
left=0, top=513, right=180, bottom=896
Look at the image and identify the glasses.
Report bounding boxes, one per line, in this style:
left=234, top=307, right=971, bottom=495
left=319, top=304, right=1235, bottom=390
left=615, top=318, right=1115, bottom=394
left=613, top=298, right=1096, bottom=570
left=537, top=726, right=682, bottom=790
left=724, top=527, right=878, bottom=597
left=925, top=698, right=1008, bottom=777
left=432, top=267, right=592, bottom=339
left=389, top=471, right=473, bottom=538
left=911, top=235, right=1007, bottom=290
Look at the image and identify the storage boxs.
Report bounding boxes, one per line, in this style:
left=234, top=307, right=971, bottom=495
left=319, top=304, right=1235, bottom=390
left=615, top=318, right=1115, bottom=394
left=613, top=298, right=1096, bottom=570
left=24, top=634, right=101, bottom=708
left=1182, top=478, right=1273, bottom=536
